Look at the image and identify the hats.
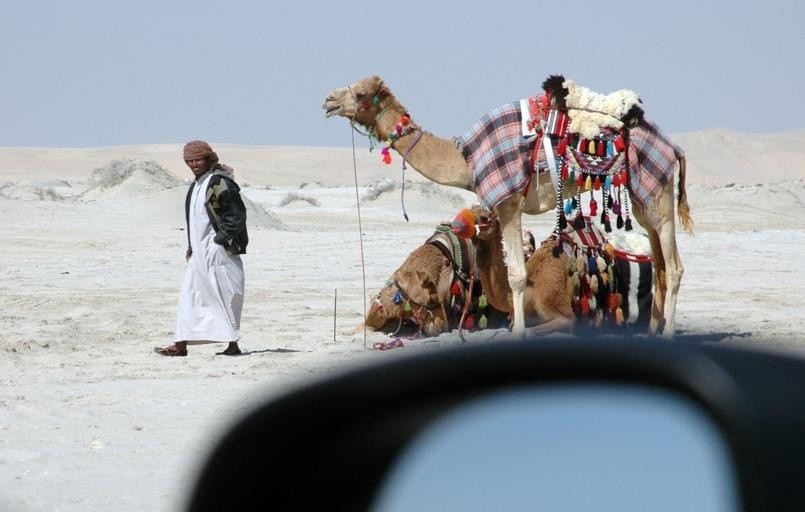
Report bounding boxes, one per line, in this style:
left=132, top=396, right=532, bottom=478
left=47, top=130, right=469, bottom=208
left=183, top=140, right=213, bottom=161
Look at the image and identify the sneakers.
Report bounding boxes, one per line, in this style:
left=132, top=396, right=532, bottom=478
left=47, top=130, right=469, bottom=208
left=155, top=347, right=187, bottom=357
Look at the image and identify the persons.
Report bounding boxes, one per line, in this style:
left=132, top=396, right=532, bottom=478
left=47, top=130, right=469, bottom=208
left=154, top=139, right=250, bottom=358
